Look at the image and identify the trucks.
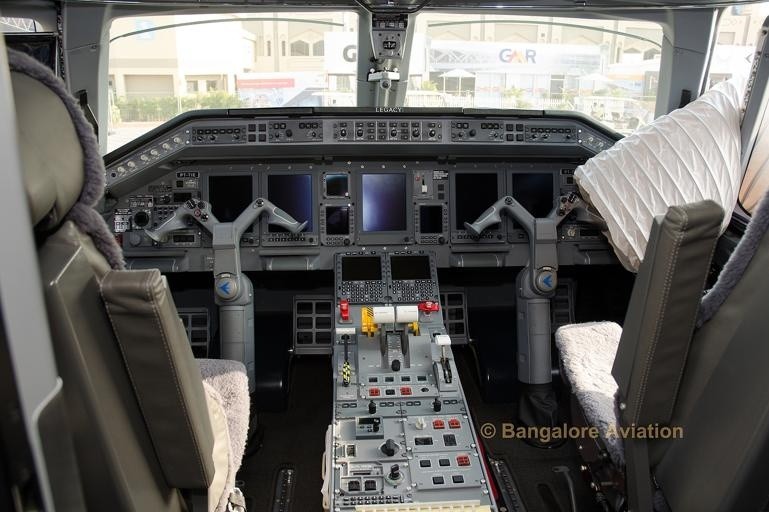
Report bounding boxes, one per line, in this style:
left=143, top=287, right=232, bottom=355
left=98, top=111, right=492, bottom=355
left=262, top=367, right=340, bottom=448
left=574, top=93, right=649, bottom=123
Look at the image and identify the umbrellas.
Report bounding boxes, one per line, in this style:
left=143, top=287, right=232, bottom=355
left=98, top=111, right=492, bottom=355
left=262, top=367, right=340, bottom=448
left=439, top=69, right=475, bottom=95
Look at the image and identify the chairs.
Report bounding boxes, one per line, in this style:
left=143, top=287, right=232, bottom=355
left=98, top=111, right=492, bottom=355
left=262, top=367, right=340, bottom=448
left=553, top=109, right=767, bottom=512
left=0, top=48, right=252, bottom=511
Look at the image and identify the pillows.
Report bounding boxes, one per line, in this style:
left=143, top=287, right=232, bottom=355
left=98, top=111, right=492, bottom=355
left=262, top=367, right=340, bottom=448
left=572, top=74, right=745, bottom=273
